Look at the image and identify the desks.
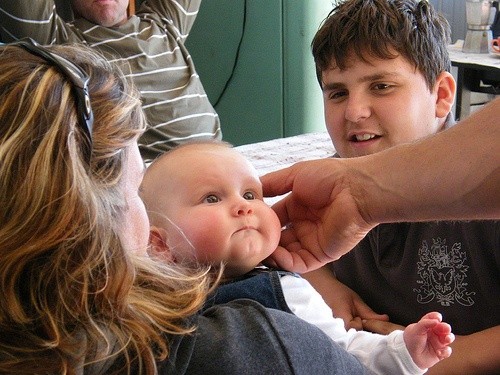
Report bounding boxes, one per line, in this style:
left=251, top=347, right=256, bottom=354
left=446, top=42, right=500, bottom=121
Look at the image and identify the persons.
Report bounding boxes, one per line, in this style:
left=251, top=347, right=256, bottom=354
left=290, top=0, right=500, bottom=374
left=256, top=97, right=500, bottom=275
left=138, top=139, right=456, bottom=375
left=0, top=38, right=377, bottom=374
left=0, top=0, right=225, bottom=164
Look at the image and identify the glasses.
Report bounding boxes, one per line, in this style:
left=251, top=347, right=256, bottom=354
left=13, top=36, right=94, bottom=170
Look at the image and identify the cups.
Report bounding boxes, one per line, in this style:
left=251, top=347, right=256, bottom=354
left=490, top=36, right=500, bottom=53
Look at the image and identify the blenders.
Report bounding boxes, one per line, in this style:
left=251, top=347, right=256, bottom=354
left=460, top=0, right=496, bottom=53
